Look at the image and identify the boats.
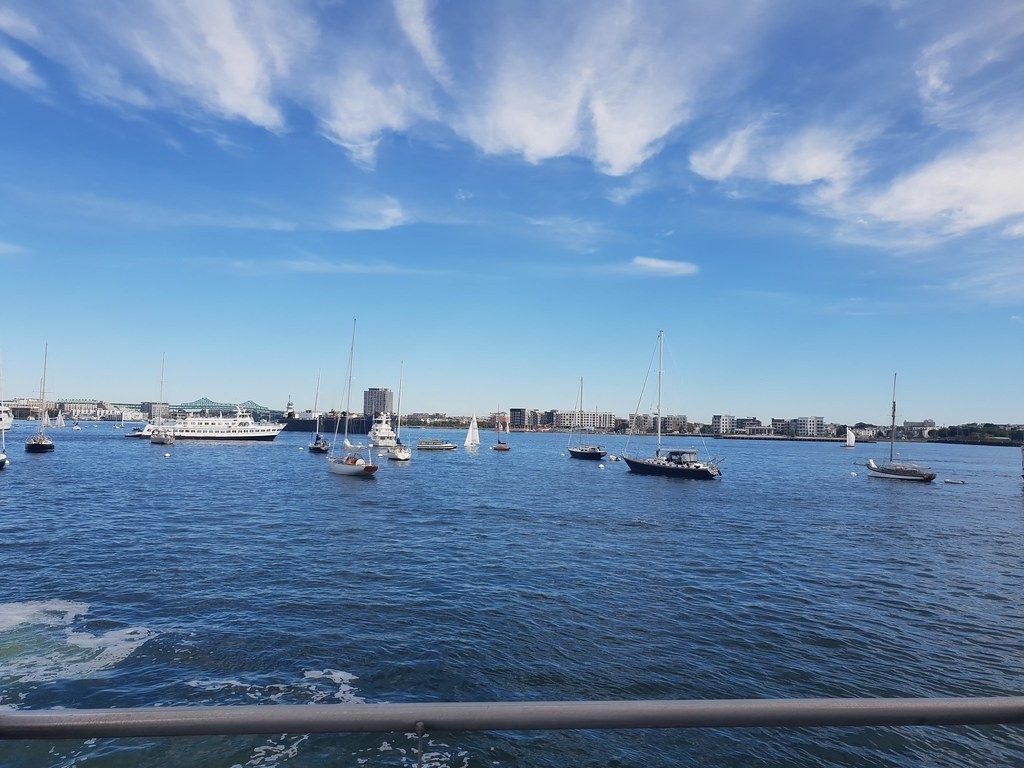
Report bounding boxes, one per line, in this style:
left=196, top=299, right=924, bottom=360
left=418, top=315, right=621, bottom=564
left=416, top=439, right=458, bottom=450
left=367, top=401, right=399, bottom=447
left=139, top=411, right=288, bottom=441
left=125, top=426, right=142, bottom=437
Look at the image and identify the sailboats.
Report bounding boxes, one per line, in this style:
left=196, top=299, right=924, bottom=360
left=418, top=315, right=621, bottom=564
left=840, top=426, right=856, bottom=448
left=463, top=412, right=481, bottom=447
left=40, top=409, right=126, bottom=431
left=25, top=342, right=56, bottom=454
left=149, top=352, right=176, bottom=444
left=324, top=315, right=379, bottom=476
left=618, top=328, right=727, bottom=480
left=387, top=359, right=413, bottom=461
left=863, top=373, right=936, bottom=482
left=0, top=392, right=15, bottom=470
left=308, top=368, right=332, bottom=454
left=565, top=375, right=608, bottom=461
left=491, top=402, right=511, bottom=451
